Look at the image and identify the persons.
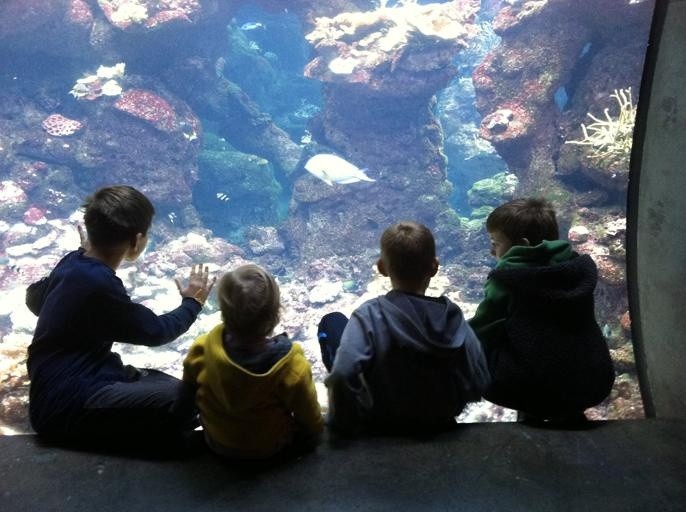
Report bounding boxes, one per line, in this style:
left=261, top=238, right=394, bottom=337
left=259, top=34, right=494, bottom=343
left=23, top=184, right=218, bottom=448
left=467, top=194, right=617, bottom=428
left=318, top=220, right=491, bottom=444
left=181, top=263, right=327, bottom=472
